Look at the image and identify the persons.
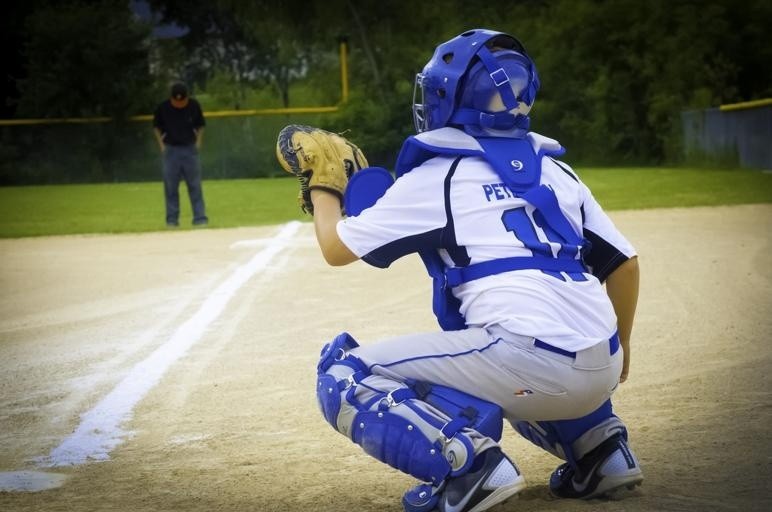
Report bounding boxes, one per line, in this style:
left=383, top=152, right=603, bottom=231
left=274, top=26, right=646, bottom=510
left=152, top=85, right=209, bottom=228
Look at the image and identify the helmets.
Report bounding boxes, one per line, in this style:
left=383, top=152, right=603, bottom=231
left=168, top=82, right=191, bottom=101
left=409, top=28, right=541, bottom=138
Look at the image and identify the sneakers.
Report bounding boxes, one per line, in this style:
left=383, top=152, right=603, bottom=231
left=435, top=445, right=527, bottom=511
left=549, top=432, right=646, bottom=502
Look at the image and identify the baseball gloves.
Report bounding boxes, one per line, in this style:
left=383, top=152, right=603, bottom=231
left=277, top=123, right=370, bottom=216
